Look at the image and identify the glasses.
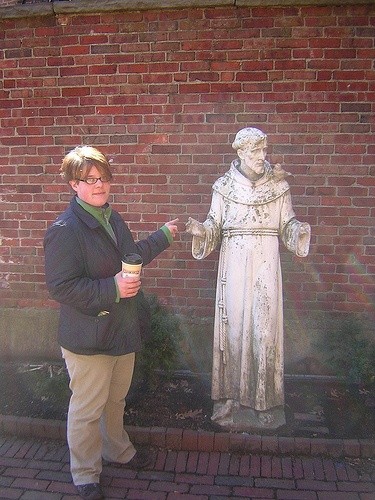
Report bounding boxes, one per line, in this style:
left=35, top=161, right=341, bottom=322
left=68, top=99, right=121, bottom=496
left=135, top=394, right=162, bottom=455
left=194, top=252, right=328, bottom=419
left=75, top=175, right=112, bottom=185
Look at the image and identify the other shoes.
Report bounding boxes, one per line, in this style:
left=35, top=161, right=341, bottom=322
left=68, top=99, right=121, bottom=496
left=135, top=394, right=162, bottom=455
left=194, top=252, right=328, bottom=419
left=103, top=449, right=154, bottom=470
left=76, top=481, right=104, bottom=500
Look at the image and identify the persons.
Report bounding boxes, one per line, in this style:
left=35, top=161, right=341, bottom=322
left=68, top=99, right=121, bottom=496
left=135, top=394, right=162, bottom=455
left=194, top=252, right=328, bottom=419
left=40, top=146, right=180, bottom=500
left=186, top=128, right=313, bottom=432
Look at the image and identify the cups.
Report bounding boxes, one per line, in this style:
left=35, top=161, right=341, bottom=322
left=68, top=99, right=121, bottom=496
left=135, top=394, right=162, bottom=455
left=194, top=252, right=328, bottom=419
left=120, top=252, right=143, bottom=279
